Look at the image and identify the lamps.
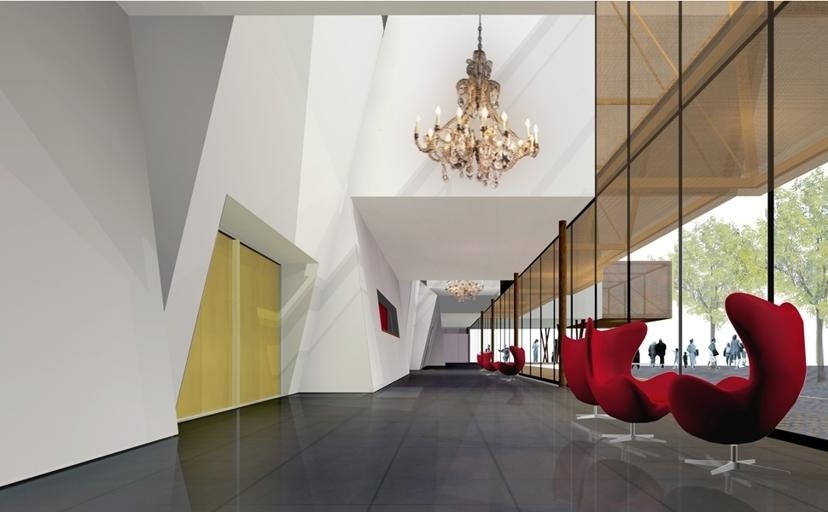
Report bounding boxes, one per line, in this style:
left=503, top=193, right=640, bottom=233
left=413, top=13, right=539, bottom=189
left=444, top=278, right=485, bottom=302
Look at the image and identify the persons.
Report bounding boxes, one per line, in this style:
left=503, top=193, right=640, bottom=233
left=532, top=339, right=539, bottom=364
left=630, top=334, right=748, bottom=370
left=499, top=344, right=510, bottom=362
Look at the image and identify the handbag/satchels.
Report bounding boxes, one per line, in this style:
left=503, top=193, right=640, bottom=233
left=712, top=348, right=718, bottom=356
left=695, top=349, right=698, bottom=356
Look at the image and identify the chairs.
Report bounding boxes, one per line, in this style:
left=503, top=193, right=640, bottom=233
left=477, top=353, right=487, bottom=372
left=494, top=346, right=525, bottom=383
left=668, top=293, right=806, bottom=475
left=561, top=335, right=616, bottom=420
left=585, top=316, right=678, bottom=444
left=480, top=351, right=500, bottom=375
